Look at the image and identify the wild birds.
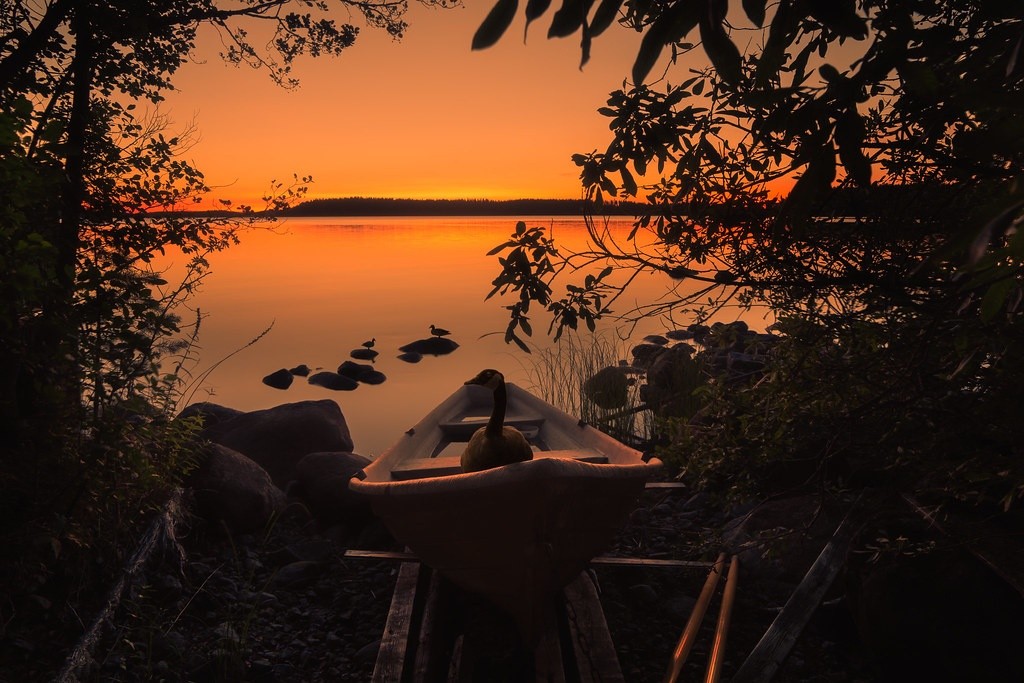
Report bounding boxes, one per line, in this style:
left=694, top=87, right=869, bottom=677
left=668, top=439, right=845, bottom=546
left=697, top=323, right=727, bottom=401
left=361, top=338, right=376, bottom=349
left=460, top=369, right=534, bottom=474
left=429, top=324, right=451, bottom=338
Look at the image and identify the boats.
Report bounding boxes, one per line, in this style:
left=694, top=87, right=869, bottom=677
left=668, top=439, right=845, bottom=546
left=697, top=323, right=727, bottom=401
left=344, top=372, right=665, bottom=647
